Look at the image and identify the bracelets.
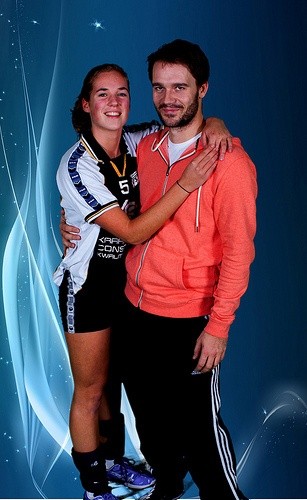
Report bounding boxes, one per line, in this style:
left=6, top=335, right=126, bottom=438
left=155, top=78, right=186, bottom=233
left=176, top=180, right=190, bottom=195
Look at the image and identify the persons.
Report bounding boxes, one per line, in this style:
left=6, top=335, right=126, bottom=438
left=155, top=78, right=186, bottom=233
left=59, top=37, right=248, bottom=500
left=55, top=63, right=232, bottom=499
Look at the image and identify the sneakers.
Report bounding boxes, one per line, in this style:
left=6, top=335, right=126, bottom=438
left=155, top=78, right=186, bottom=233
left=84, top=483, right=118, bottom=500
left=105, top=457, right=156, bottom=489
